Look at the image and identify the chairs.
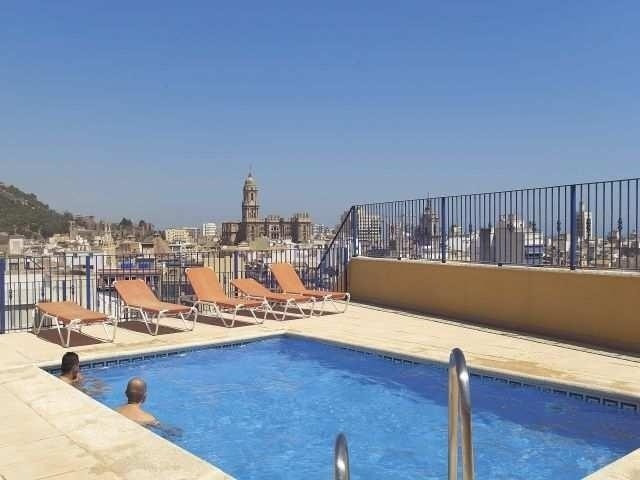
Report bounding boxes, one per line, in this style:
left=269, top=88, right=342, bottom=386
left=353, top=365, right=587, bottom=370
left=110, top=279, right=199, bottom=333
left=30, top=299, right=118, bottom=346
left=178, top=265, right=270, bottom=329
left=230, top=275, right=317, bottom=321
left=269, top=261, right=351, bottom=318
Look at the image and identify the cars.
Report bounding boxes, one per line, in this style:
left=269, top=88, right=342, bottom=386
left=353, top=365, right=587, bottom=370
left=315, top=272, right=331, bottom=289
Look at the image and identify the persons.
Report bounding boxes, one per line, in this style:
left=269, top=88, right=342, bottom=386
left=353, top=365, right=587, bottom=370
left=56, top=351, right=82, bottom=385
left=110, top=377, right=177, bottom=436
